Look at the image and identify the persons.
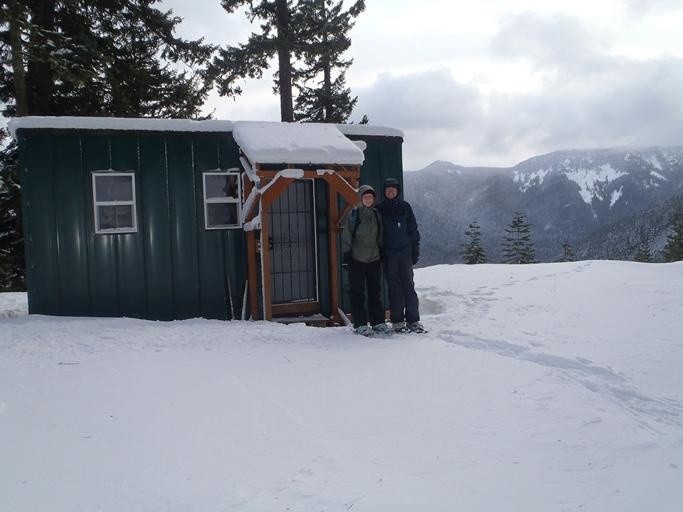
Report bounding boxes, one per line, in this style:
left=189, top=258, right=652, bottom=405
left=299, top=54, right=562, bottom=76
left=340, top=183, right=395, bottom=337
left=372, top=176, right=426, bottom=334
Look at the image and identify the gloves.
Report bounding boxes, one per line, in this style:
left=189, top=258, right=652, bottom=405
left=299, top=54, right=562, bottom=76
left=413, top=246, right=419, bottom=265
left=343, top=251, right=352, bottom=272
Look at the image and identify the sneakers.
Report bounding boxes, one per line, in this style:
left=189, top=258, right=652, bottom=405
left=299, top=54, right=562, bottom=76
left=393, top=322, right=411, bottom=334
left=373, top=322, right=393, bottom=336
left=356, top=325, right=374, bottom=337
left=407, top=321, right=428, bottom=333
